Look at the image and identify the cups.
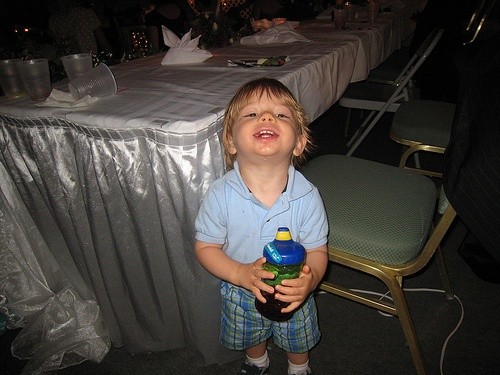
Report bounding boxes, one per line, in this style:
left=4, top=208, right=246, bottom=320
left=0, top=59, right=23, bottom=105
left=18, top=59, right=51, bottom=98
left=68, top=64, right=117, bottom=100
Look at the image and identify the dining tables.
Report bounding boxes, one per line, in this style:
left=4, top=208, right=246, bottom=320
left=0, top=0, right=430, bottom=375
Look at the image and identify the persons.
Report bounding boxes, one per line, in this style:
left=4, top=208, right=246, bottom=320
left=47, top=0, right=190, bottom=56
left=194, top=77, right=329, bottom=375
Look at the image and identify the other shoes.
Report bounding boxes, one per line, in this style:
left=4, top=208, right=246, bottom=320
left=236, top=360, right=271, bottom=375
left=288, top=369, right=312, bottom=375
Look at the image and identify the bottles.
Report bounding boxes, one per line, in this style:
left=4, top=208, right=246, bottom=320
left=255, top=227, right=307, bottom=321
left=58, top=53, right=93, bottom=79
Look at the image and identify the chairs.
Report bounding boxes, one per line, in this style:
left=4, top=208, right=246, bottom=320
left=338, top=27, right=444, bottom=154
left=389, top=100, right=459, bottom=169
left=364, top=0, right=500, bottom=93
left=298, top=154, right=458, bottom=374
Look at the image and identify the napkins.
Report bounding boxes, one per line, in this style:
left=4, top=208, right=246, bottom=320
left=240, top=27, right=313, bottom=46
left=160, top=25, right=213, bottom=65
